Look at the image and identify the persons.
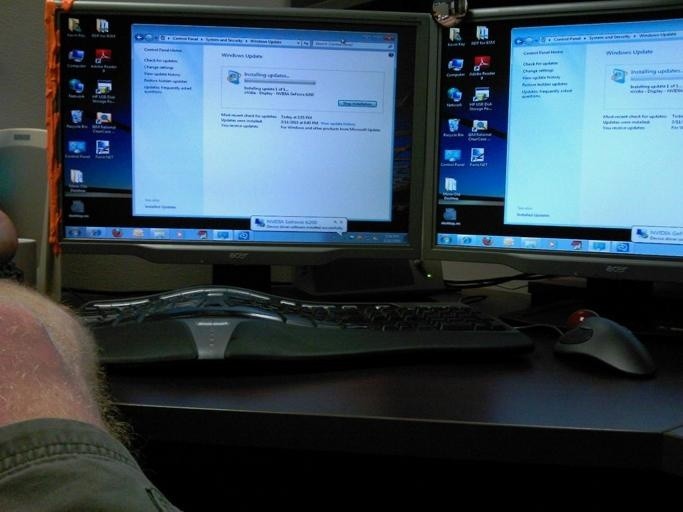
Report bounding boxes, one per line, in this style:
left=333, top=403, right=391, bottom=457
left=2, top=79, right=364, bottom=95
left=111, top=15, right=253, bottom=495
left=1, top=278, right=184, bottom=512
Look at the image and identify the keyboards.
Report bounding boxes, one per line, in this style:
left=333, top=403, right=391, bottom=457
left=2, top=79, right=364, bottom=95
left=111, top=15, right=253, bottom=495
left=75, top=282, right=536, bottom=361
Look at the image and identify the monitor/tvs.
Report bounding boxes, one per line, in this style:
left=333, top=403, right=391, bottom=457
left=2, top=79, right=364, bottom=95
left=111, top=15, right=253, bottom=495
left=46, top=0, right=431, bottom=291
left=419, top=1, right=682, bottom=339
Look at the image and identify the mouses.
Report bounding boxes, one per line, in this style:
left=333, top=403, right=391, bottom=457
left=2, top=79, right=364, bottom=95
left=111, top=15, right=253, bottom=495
left=552, top=314, right=659, bottom=381
